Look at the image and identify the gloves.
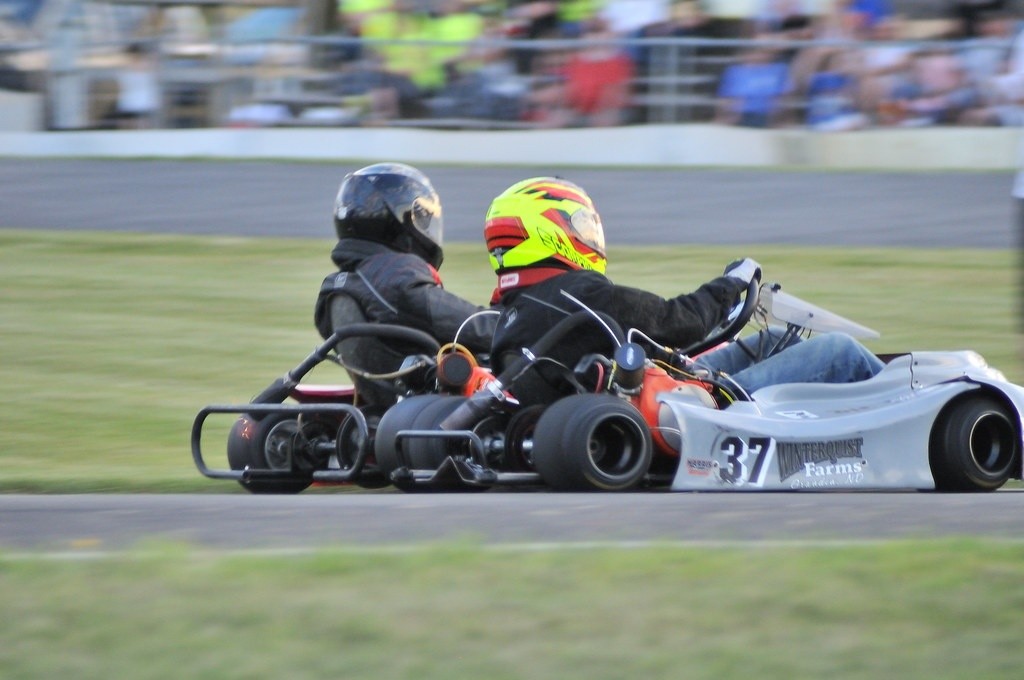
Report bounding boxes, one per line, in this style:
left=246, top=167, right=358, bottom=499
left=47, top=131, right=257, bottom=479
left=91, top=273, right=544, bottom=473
left=722, top=257, right=761, bottom=293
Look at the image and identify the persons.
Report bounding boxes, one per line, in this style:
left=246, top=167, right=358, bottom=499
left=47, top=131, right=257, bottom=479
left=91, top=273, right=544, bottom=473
left=1, top=1, right=1023, bottom=130
left=312, top=161, right=507, bottom=406
left=483, top=176, right=886, bottom=401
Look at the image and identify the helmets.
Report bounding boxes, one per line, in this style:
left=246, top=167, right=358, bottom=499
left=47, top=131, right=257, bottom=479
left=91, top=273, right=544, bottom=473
left=333, top=163, right=444, bottom=271
left=484, top=176, right=606, bottom=275
